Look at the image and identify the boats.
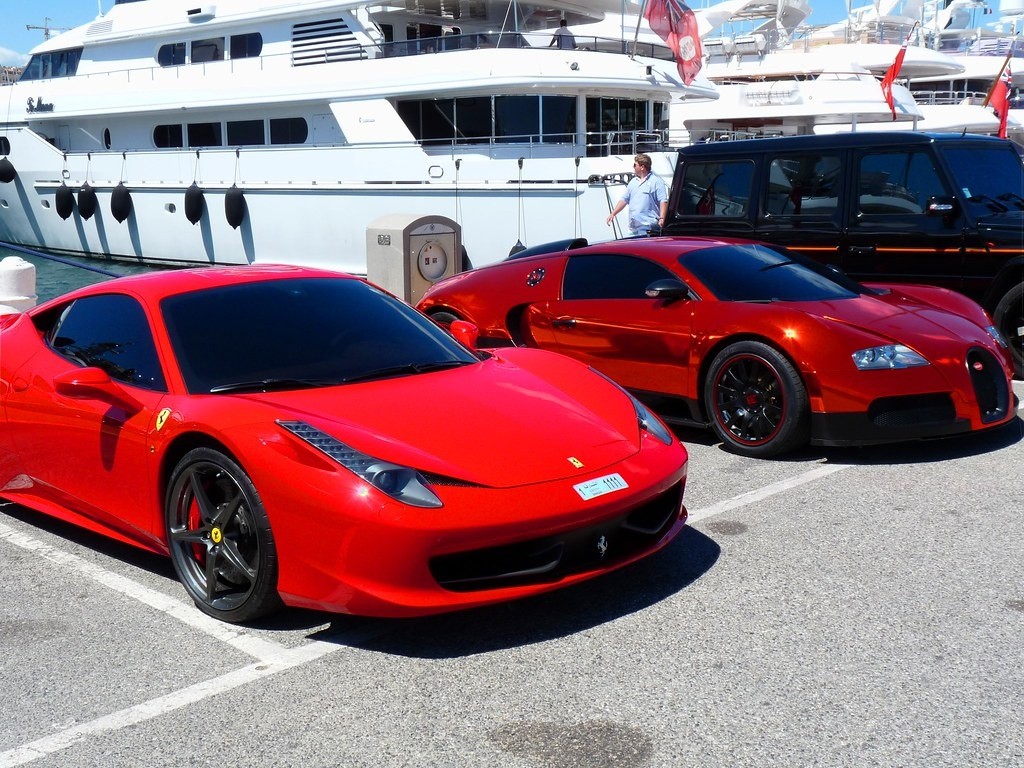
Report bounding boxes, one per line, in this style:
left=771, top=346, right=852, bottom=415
left=638, top=0, right=1024, bottom=155
left=0, top=0, right=692, bottom=303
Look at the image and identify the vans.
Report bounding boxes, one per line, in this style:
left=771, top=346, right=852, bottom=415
left=663, top=130, right=1024, bottom=382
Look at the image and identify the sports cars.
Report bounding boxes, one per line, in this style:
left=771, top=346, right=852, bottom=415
left=419, top=234, right=1018, bottom=456
left=2, top=261, right=693, bottom=628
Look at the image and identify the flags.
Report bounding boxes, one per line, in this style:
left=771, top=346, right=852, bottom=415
left=989, top=60, right=1013, bottom=139
left=643, top=0, right=702, bottom=86
left=880, top=26, right=915, bottom=122
left=696, top=186, right=715, bottom=215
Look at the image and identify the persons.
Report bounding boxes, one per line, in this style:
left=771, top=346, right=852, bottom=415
left=548, top=18, right=577, bottom=50
left=606, top=153, right=669, bottom=237
left=479, top=36, right=489, bottom=45
